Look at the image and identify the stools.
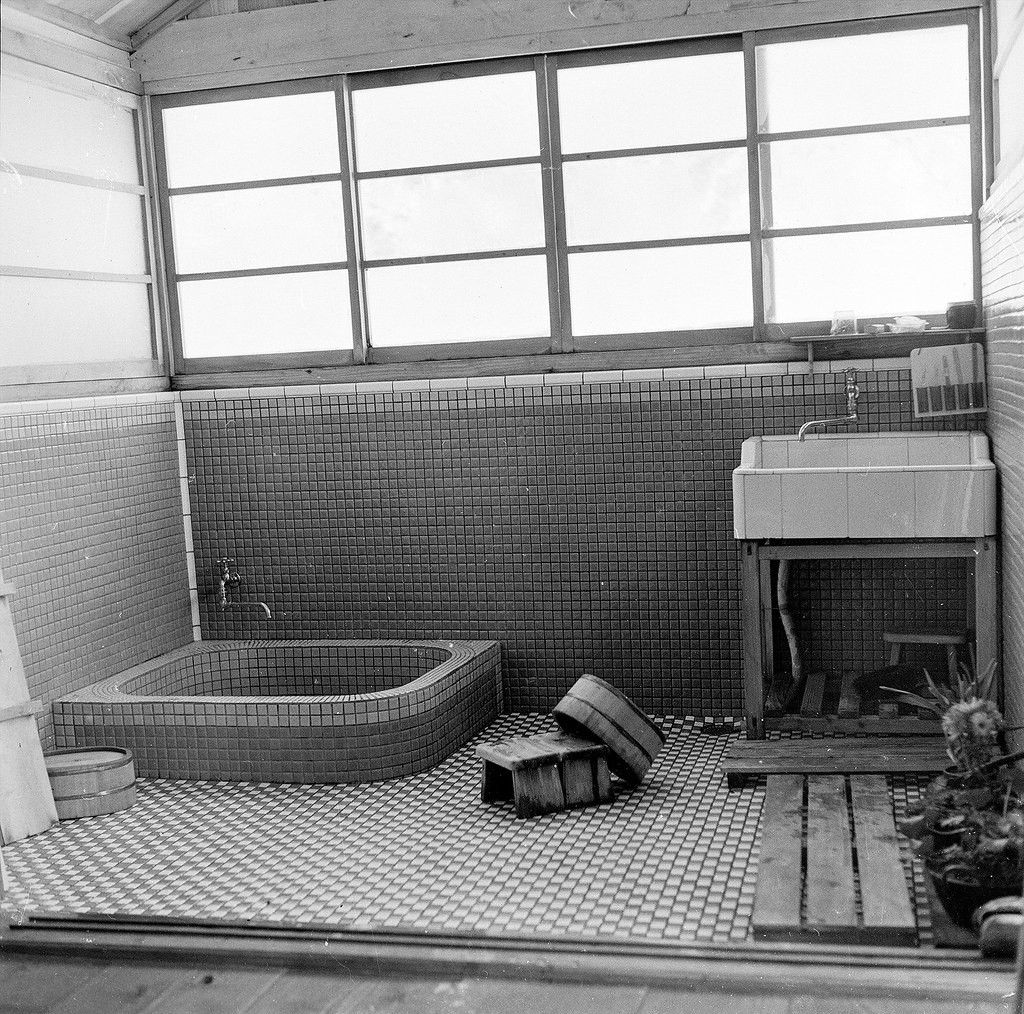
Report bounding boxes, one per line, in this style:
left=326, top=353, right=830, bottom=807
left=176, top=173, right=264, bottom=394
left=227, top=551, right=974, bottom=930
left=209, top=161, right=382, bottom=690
left=473, top=732, right=611, bottom=819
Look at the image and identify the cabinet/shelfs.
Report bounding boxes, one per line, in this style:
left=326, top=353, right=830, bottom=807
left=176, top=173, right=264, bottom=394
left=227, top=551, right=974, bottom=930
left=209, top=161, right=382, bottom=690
left=789, top=324, right=984, bottom=369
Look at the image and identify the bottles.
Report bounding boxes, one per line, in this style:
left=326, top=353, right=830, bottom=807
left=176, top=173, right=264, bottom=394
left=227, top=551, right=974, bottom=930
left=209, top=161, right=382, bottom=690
left=946, top=300, right=977, bottom=329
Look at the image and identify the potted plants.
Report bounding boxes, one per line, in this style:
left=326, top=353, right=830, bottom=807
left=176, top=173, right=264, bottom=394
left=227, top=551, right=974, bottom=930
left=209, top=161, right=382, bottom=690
left=885, top=642, right=1024, bottom=939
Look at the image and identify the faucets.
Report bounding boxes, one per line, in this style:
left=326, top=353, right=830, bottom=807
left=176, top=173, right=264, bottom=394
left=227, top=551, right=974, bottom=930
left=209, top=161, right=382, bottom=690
left=797, top=414, right=862, bottom=442
left=220, top=597, right=274, bottom=623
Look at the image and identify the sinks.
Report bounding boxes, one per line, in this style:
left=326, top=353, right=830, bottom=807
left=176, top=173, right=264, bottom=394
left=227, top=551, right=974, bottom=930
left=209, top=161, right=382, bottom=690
left=731, top=430, right=998, bottom=541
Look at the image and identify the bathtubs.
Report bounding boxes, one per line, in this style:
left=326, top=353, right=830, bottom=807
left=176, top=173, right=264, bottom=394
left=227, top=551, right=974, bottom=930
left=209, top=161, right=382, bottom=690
left=50, top=639, right=507, bottom=786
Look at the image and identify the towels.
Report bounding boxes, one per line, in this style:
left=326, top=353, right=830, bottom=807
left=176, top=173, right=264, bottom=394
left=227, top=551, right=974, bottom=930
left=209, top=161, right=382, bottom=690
left=909, top=342, right=989, bottom=420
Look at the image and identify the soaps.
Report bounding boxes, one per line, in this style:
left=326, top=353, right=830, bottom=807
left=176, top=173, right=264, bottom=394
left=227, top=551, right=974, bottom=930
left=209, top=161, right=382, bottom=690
left=863, top=323, right=885, bottom=335
